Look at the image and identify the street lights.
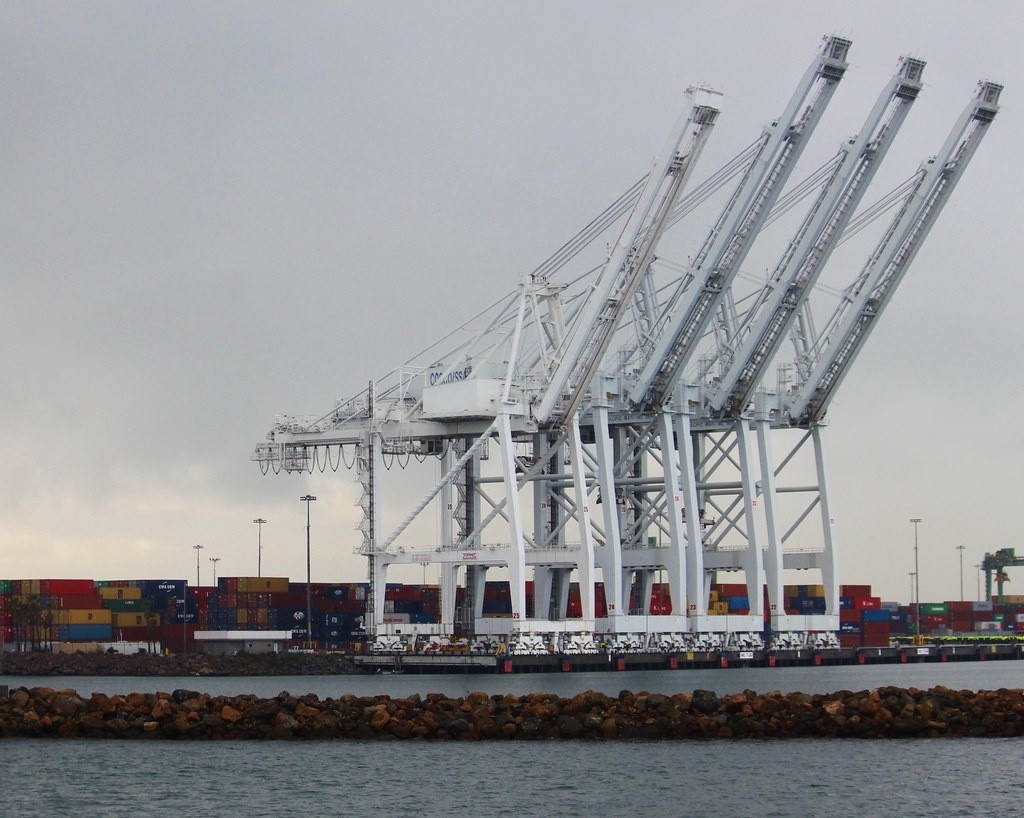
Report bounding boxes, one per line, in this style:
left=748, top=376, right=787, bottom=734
left=254, top=517, right=267, bottom=578
left=973, top=564, right=983, bottom=601
left=194, top=544, right=204, bottom=587
left=300, top=494, right=318, bottom=646
left=208, top=556, right=221, bottom=587
left=955, top=544, right=967, bottom=600
left=911, top=517, right=921, bottom=635
